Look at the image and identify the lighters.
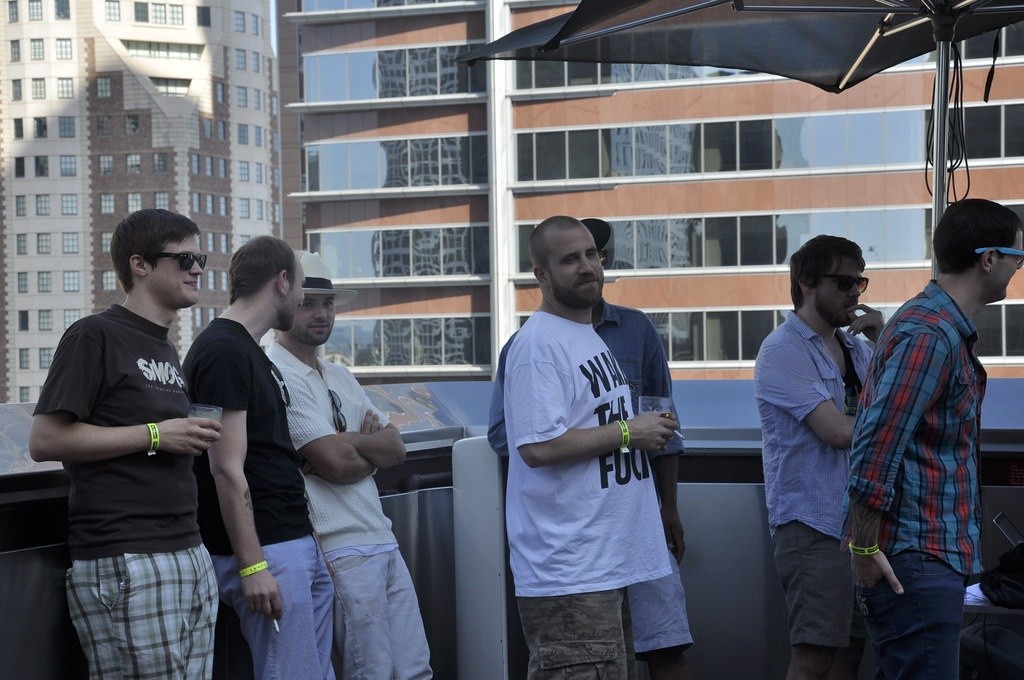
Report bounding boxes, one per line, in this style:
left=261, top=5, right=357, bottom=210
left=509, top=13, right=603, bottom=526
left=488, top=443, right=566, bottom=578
left=661, top=412, right=673, bottom=441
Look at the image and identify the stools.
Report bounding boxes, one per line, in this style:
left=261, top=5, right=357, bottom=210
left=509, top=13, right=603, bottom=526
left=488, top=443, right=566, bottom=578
left=959, top=620, right=1024, bottom=680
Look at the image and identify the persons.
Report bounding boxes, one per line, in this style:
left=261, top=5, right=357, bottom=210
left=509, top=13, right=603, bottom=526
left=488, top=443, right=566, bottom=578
left=265, top=252, right=434, bottom=680
left=182, top=235, right=336, bottom=680
left=755, top=235, right=885, bottom=680
left=488, top=218, right=694, bottom=680
left=506, top=215, right=680, bottom=680
left=29, top=208, right=222, bottom=680
left=840, top=199, right=1024, bottom=680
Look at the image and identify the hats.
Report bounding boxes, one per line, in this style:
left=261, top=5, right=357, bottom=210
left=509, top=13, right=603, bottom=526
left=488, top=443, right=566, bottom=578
left=580, top=219, right=610, bottom=250
left=296, top=251, right=358, bottom=306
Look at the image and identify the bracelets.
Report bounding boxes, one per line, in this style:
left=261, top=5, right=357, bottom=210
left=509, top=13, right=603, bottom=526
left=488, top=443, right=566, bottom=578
left=849, top=541, right=879, bottom=556
left=147, top=423, right=159, bottom=458
left=618, top=418, right=630, bottom=455
left=372, top=467, right=378, bottom=476
left=239, top=561, right=268, bottom=578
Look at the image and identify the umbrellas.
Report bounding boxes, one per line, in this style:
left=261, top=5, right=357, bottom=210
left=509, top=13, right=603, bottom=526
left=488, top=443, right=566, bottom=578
left=454, top=0, right=1024, bottom=281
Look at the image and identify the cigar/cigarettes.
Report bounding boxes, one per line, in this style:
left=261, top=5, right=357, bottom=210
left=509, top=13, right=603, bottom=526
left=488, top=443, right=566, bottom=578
left=270, top=614, right=281, bottom=634
left=671, top=427, right=685, bottom=440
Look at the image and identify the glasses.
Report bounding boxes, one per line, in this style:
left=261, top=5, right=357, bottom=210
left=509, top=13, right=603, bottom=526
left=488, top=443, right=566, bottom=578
left=598, top=249, right=608, bottom=266
left=975, top=247, right=1024, bottom=269
left=820, top=273, right=869, bottom=293
left=156, top=252, right=207, bottom=271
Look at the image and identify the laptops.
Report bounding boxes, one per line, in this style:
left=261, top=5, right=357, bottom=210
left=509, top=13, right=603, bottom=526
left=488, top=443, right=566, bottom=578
left=992, top=512, right=1024, bottom=547
